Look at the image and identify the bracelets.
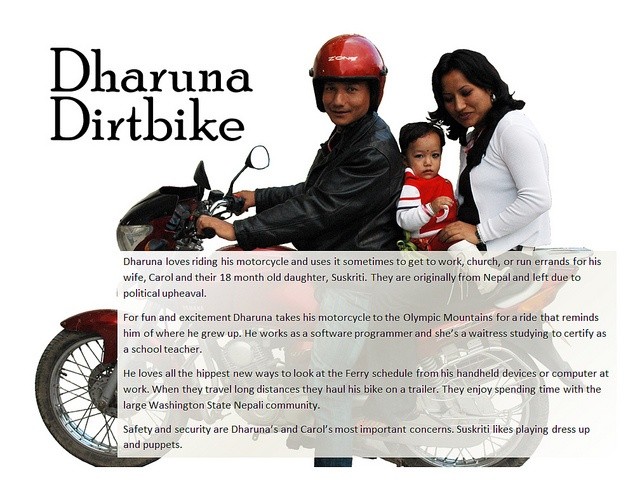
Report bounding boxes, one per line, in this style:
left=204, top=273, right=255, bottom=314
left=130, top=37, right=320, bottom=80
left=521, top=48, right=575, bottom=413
left=475, top=226, right=486, bottom=246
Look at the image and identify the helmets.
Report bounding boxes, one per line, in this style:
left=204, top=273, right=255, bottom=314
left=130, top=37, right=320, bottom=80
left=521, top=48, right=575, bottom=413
left=310, top=34, right=387, bottom=113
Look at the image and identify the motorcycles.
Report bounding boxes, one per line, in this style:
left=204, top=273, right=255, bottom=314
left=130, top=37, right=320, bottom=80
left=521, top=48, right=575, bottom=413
left=34, top=143, right=595, bottom=468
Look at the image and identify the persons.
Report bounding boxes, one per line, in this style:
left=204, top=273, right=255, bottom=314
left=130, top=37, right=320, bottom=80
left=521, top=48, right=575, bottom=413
left=427, top=49, right=551, bottom=315
left=196, top=34, right=405, bottom=466
left=395, top=122, right=455, bottom=251
